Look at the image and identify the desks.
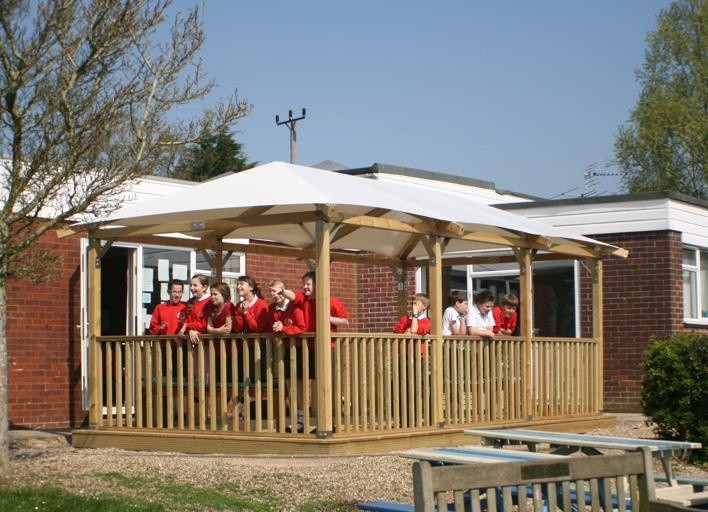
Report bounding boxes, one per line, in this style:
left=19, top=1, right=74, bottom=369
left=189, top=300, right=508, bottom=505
left=389, top=428, right=708, bottom=512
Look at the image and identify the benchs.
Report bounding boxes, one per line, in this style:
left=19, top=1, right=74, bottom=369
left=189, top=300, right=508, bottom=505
left=359, top=499, right=439, bottom=512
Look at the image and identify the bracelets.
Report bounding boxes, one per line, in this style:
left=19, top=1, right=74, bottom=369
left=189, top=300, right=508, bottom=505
left=411, top=314, right=418, bottom=318
left=278, top=286, right=284, bottom=295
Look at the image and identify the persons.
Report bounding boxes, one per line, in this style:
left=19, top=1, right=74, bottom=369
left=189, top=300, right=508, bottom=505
left=265, top=279, right=307, bottom=433
left=269, top=271, right=350, bottom=434
left=440, top=292, right=470, bottom=419
left=392, top=294, right=430, bottom=423
left=149, top=279, right=189, bottom=422
left=232, top=275, right=270, bottom=418
left=178, top=272, right=212, bottom=424
left=460, top=290, right=496, bottom=414
left=490, top=293, right=519, bottom=414
left=205, top=280, right=241, bottom=420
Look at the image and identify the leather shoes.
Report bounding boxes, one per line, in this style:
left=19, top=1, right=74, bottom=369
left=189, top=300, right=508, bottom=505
left=285, top=421, right=336, bottom=434
